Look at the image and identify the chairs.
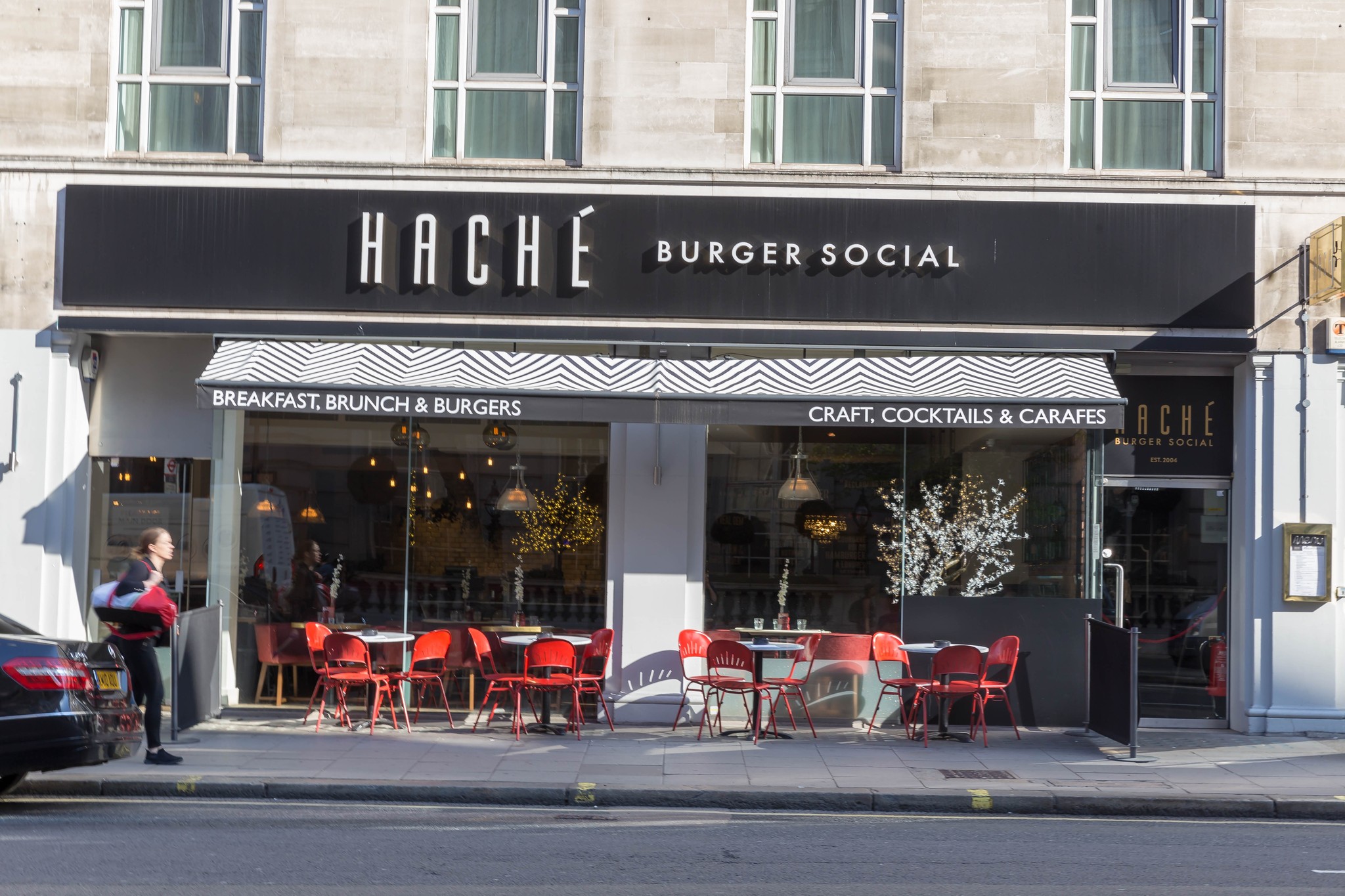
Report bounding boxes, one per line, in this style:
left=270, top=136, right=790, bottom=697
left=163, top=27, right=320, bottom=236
left=908, top=644, right=989, bottom=747
left=946, top=635, right=1022, bottom=741
left=672, top=628, right=873, bottom=746
left=868, top=631, right=940, bottom=739
left=250, top=623, right=616, bottom=742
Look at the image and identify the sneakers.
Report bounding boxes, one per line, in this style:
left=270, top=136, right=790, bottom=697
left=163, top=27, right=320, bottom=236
left=143, top=748, right=183, bottom=765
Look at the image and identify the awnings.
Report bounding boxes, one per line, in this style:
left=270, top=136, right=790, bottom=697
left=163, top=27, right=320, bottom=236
left=195, top=341, right=1127, bottom=431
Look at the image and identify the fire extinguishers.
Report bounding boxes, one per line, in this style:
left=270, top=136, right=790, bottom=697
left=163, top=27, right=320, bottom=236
left=1208, top=637, right=1228, bottom=696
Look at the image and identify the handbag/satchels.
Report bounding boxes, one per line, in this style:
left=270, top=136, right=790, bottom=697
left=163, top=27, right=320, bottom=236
left=90, top=560, right=180, bottom=642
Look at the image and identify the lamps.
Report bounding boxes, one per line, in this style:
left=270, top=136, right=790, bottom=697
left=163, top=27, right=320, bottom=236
left=777, top=427, right=824, bottom=501
left=390, top=416, right=432, bottom=498
left=368, top=452, right=377, bottom=468
left=465, top=494, right=472, bottom=509
left=482, top=419, right=539, bottom=512
left=244, top=412, right=284, bottom=519
left=292, top=446, right=329, bottom=526
left=458, top=454, right=468, bottom=481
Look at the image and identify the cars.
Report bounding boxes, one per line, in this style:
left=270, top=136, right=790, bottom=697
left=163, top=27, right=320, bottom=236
left=0, top=615, right=145, bottom=795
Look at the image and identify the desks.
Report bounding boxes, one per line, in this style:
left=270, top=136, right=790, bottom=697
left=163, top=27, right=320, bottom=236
left=406, top=629, right=433, bottom=698
left=898, top=641, right=991, bottom=744
left=732, top=627, right=831, bottom=658
left=500, top=634, right=593, bottom=736
left=337, top=630, right=414, bottom=729
left=480, top=624, right=561, bottom=686
left=291, top=622, right=370, bottom=707
left=717, top=641, right=805, bottom=740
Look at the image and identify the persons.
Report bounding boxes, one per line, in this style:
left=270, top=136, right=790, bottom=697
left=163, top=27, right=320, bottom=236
left=101, top=527, right=183, bottom=765
left=289, top=543, right=326, bottom=624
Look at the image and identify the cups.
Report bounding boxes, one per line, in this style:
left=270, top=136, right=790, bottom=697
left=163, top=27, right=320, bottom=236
left=754, top=618, right=764, bottom=630
left=335, top=613, right=344, bottom=624
left=450, top=610, right=458, bottom=621
left=529, top=616, right=539, bottom=626
left=473, top=611, right=481, bottom=622
left=773, top=619, right=782, bottom=630
left=797, top=619, right=807, bottom=630
left=317, top=612, right=323, bottom=622
